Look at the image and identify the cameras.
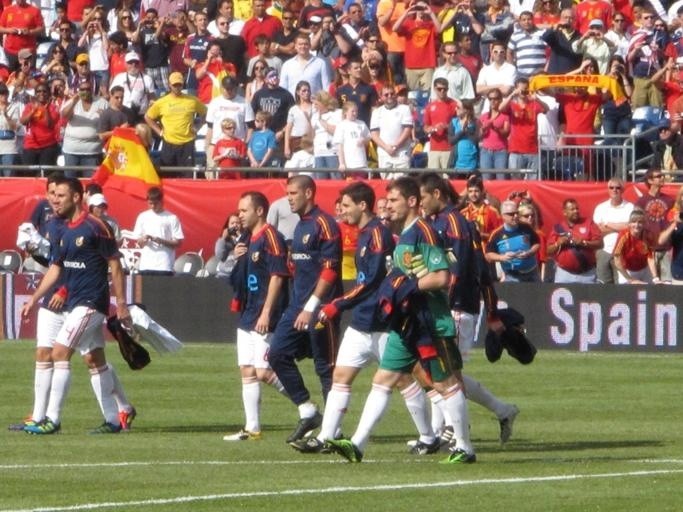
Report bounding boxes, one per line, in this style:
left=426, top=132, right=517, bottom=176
left=512, top=191, right=521, bottom=196
left=614, top=64, right=624, bottom=76
left=144, top=20, right=155, bottom=24
left=561, top=24, right=571, bottom=29
left=212, top=50, right=223, bottom=57
left=414, top=6, right=425, bottom=10
left=78, top=91, right=91, bottom=98
left=589, top=32, right=600, bottom=36
left=521, top=90, right=529, bottom=95
left=228, top=227, right=237, bottom=235
left=53, top=86, right=58, bottom=97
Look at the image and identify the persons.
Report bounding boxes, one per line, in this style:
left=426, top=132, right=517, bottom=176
left=293, top=181, right=442, bottom=454
left=222, top=191, right=291, bottom=442
left=0, top=0, right=683, bottom=287
left=268, top=174, right=342, bottom=441
left=407, top=172, right=471, bottom=447
left=324, top=178, right=476, bottom=466
left=443, top=178, right=520, bottom=444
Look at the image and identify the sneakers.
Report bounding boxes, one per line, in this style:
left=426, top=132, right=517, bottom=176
left=286, top=412, right=361, bottom=463
left=224, top=428, right=261, bottom=442
left=8, top=416, right=60, bottom=434
left=407, top=424, right=475, bottom=464
left=498, top=405, right=518, bottom=441
left=89, top=407, right=135, bottom=434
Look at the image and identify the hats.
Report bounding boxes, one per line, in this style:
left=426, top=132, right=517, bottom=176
left=18, top=48, right=32, bottom=58
left=588, top=19, right=603, bottom=26
left=222, top=76, right=235, bottom=88
left=76, top=53, right=89, bottom=64
left=87, top=193, right=107, bottom=211
left=125, top=52, right=139, bottom=61
left=168, top=72, right=183, bottom=84
left=656, top=119, right=670, bottom=130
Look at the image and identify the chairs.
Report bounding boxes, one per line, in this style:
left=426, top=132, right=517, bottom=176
left=0, top=249, right=23, bottom=273
left=173, top=252, right=205, bottom=277
left=205, top=256, right=220, bottom=276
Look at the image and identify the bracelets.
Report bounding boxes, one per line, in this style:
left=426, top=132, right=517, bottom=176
left=304, top=294, right=321, bottom=312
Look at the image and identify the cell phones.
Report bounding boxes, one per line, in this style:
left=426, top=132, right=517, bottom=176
left=24, top=61, right=28, bottom=66
